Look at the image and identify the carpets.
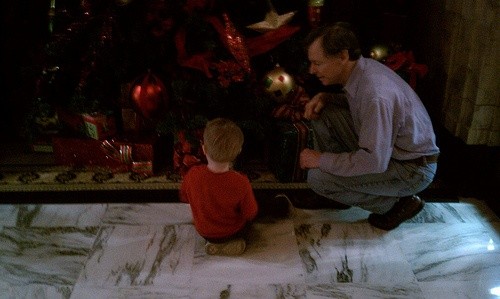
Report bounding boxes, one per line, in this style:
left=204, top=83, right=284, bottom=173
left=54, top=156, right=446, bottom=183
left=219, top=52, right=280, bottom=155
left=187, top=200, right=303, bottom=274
left=0, top=149, right=307, bottom=192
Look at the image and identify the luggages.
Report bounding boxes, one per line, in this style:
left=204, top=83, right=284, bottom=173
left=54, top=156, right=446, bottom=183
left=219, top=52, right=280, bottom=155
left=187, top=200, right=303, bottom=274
left=268, top=120, right=315, bottom=183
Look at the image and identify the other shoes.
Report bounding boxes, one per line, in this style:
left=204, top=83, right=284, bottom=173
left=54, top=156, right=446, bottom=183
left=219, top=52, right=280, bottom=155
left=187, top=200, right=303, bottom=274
left=366, top=195, right=423, bottom=231
left=206, top=238, right=247, bottom=256
